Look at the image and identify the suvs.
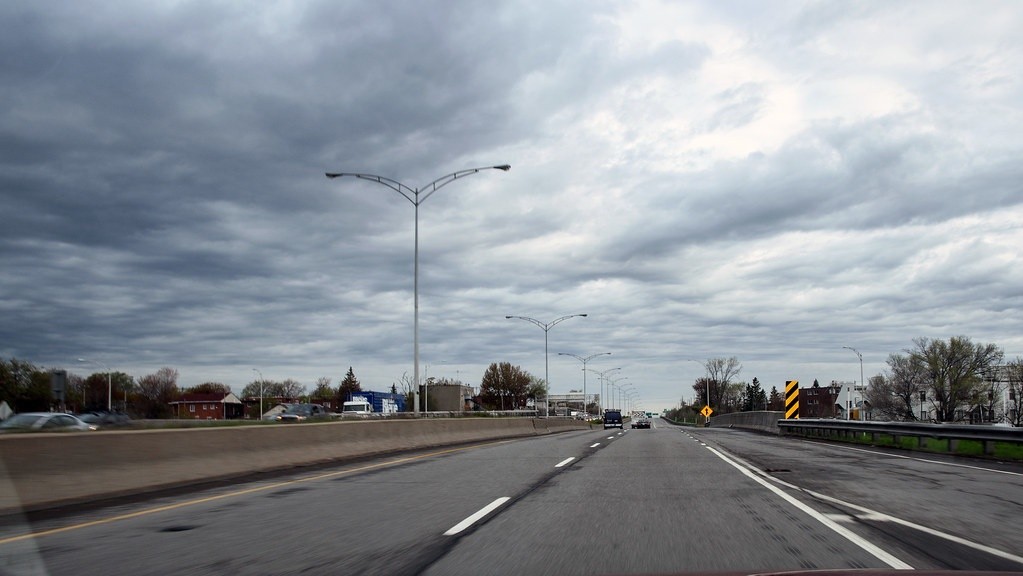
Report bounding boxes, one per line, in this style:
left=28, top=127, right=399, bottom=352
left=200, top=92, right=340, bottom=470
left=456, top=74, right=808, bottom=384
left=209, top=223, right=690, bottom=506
left=277, top=404, right=324, bottom=422
left=75, top=411, right=132, bottom=429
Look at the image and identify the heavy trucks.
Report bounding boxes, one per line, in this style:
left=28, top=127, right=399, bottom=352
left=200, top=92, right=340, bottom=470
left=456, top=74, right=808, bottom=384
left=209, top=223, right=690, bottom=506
left=341, top=392, right=406, bottom=418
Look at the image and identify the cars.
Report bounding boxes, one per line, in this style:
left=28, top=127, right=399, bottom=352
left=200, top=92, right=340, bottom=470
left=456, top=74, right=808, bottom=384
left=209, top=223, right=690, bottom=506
left=579, top=415, right=602, bottom=421
left=637, top=419, right=650, bottom=429
left=0, top=412, right=97, bottom=433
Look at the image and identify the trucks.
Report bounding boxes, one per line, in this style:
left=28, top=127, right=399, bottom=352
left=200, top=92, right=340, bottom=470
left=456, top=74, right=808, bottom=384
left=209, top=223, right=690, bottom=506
left=631, top=412, right=648, bottom=428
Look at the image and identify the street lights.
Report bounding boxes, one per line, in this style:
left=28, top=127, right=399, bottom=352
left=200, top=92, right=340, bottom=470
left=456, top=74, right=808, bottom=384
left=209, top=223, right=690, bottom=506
left=688, top=359, right=709, bottom=423
left=424, top=359, right=447, bottom=414
left=253, top=368, right=263, bottom=421
left=843, top=346, right=864, bottom=421
left=326, top=164, right=510, bottom=416
left=581, top=367, right=619, bottom=415
left=599, top=377, right=640, bottom=415
left=506, top=313, right=589, bottom=415
left=558, top=352, right=611, bottom=416
left=78, top=358, right=111, bottom=411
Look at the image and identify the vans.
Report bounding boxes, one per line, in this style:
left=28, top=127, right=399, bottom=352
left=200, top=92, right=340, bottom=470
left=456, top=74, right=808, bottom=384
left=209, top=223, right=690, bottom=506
left=603, top=411, right=623, bottom=429
